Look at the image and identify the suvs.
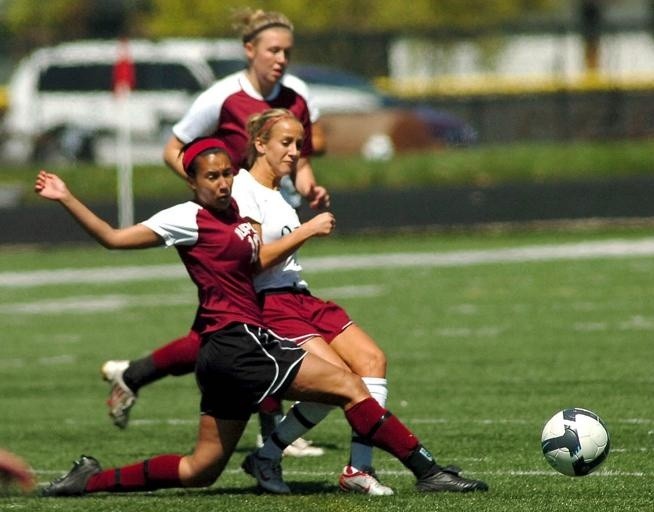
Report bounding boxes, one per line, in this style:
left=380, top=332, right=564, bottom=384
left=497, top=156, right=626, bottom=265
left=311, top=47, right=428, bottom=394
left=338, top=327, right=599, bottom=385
left=7, top=38, right=393, bottom=167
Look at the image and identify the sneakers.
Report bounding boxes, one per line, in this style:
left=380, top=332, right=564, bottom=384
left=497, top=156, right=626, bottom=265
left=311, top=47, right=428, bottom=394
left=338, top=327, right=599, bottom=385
left=256, top=433, right=325, bottom=457
left=241, top=448, right=291, bottom=493
left=42, top=455, right=101, bottom=497
left=100, top=359, right=138, bottom=429
left=415, top=464, right=488, bottom=492
left=338, top=466, right=394, bottom=496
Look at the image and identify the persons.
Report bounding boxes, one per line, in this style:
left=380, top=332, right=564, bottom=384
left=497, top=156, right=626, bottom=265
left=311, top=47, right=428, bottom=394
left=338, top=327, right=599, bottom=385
left=33, top=137, right=493, bottom=497
left=0, top=450, right=37, bottom=493
left=96, top=8, right=331, bottom=456
left=224, top=105, right=394, bottom=498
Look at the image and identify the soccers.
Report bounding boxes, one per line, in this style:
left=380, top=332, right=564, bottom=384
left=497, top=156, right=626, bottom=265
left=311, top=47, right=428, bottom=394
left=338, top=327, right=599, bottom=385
left=541, top=408, right=609, bottom=477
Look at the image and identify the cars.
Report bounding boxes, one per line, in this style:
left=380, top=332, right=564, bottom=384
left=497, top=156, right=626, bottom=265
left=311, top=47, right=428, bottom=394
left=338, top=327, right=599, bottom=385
left=288, top=64, right=476, bottom=146
